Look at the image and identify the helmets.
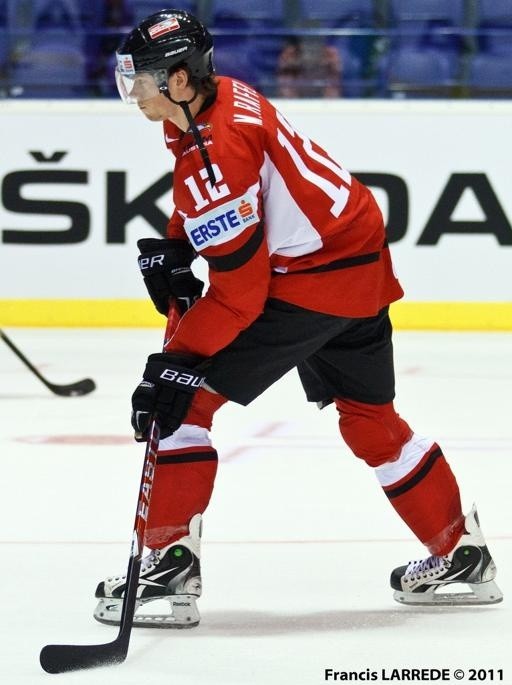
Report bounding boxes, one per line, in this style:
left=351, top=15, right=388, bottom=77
left=116, top=8, right=215, bottom=83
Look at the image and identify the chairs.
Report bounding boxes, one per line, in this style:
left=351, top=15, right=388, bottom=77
left=0, top=0, right=510, bottom=101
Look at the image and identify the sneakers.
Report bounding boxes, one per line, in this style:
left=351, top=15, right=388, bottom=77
left=388, top=509, right=498, bottom=594
left=93, top=514, right=206, bottom=602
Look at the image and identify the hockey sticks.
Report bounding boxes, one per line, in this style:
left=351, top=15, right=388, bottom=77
left=0, top=331, right=96, bottom=397
left=40, top=296, right=180, bottom=673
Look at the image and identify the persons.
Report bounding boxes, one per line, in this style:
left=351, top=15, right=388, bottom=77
left=95, top=8, right=497, bottom=600
left=273, top=21, right=344, bottom=98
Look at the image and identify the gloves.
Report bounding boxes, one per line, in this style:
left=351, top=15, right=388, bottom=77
left=129, top=349, right=217, bottom=446
left=135, top=238, right=204, bottom=318
